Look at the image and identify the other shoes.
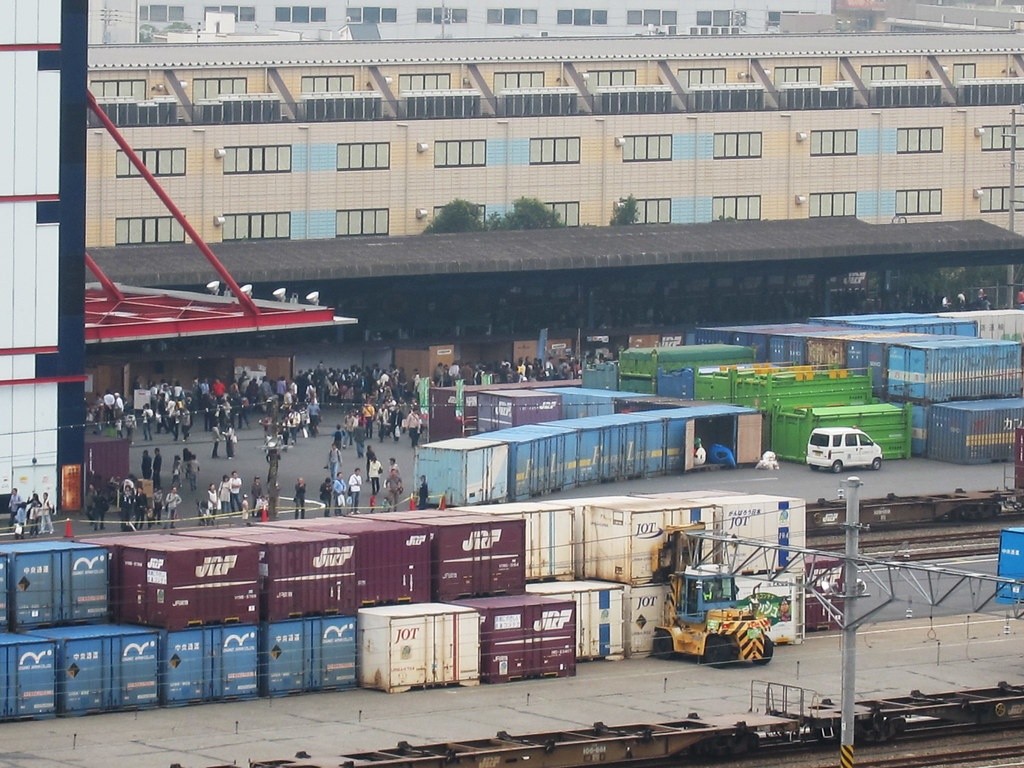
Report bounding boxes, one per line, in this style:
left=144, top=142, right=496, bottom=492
left=49, top=528, right=54, bottom=534
left=38, top=530, right=46, bottom=534
left=351, top=510, right=360, bottom=514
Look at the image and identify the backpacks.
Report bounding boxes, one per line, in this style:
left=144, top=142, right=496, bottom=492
left=146, top=413, right=155, bottom=423
left=47, top=501, right=54, bottom=513
left=102, top=501, right=108, bottom=511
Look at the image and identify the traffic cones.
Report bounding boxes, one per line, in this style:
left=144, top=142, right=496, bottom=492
left=261, top=509, right=270, bottom=521
left=439, top=495, right=446, bottom=511
left=410, top=498, right=416, bottom=510
left=63, top=517, right=75, bottom=538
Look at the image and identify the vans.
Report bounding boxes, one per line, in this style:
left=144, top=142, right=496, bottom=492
left=805, top=427, right=884, bottom=471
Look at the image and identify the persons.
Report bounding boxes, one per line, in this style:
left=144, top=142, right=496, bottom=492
left=703, top=582, right=714, bottom=602
left=1017, top=288, right=1024, bottom=304
left=957, top=293, right=966, bottom=312
left=7, top=354, right=615, bottom=540
left=942, top=296, right=952, bottom=312
left=976, top=288, right=991, bottom=310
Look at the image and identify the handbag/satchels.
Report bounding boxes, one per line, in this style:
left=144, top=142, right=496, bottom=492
left=165, top=504, right=168, bottom=512
left=378, top=468, right=383, bottom=474
left=395, top=426, right=400, bottom=437
left=231, top=435, right=237, bottom=443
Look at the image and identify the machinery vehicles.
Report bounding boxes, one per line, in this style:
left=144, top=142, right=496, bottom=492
left=652, top=562, right=773, bottom=667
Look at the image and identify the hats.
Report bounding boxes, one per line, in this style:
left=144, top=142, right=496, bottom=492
left=244, top=494, right=248, bottom=497
left=115, top=393, right=120, bottom=396
